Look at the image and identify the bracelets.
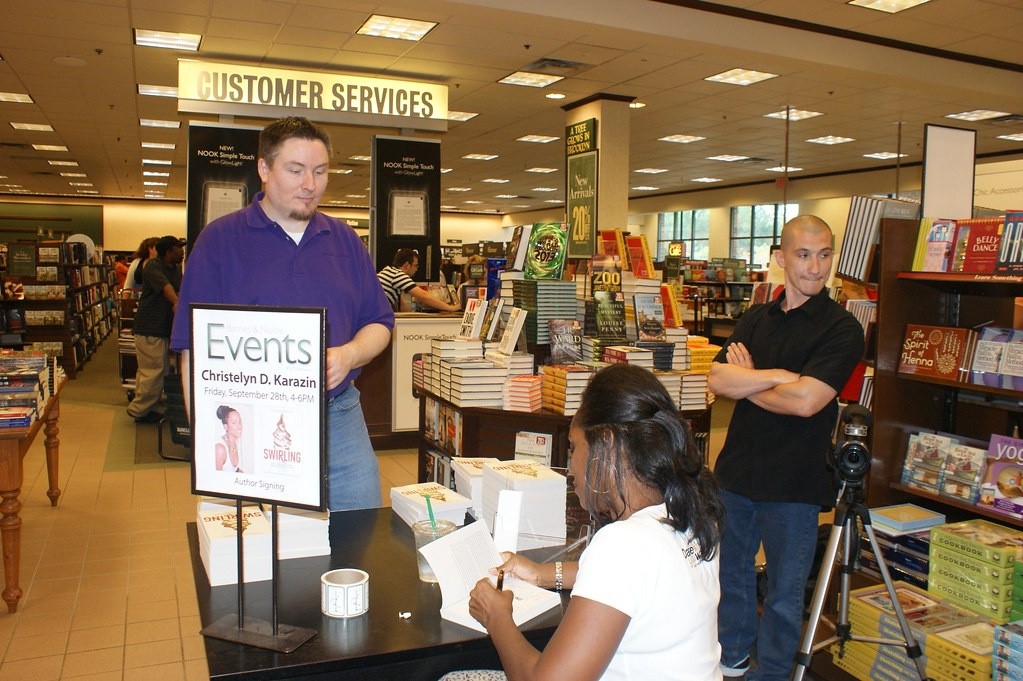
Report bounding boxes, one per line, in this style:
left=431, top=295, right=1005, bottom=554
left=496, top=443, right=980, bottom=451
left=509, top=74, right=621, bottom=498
left=556, top=561, right=563, bottom=589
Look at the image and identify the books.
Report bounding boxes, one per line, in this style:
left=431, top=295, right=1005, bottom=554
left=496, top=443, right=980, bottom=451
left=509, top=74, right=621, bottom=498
left=859, top=375, right=874, bottom=411
left=23, top=246, right=136, bottom=366
left=836, top=195, right=919, bottom=284
left=846, top=299, right=879, bottom=341
left=388, top=455, right=567, bottom=552
left=0, top=350, right=65, bottom=428
left=853, top=503, right=1023, bottom=681
left=418, top=518, right=562, bottom=636
left=910, top=211, right=1023, bottom=274
left=412, top=221, right=725, bottom=412
left=902, top=430, right=1023, bottom=519
left=196, top=498, right=332, bottom=587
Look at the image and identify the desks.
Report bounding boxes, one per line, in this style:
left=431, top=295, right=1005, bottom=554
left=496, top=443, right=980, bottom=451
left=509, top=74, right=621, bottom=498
left=0, top=375, right=68, bottom=614
left=187, top=492, right=620, bottom=681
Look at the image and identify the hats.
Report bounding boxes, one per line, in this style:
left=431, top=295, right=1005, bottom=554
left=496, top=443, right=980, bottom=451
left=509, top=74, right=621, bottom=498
left=155, top=236, right=189, bottom=254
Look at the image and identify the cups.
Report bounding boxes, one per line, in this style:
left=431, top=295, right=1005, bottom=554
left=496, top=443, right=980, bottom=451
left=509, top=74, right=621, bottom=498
left=412, top=519, right=457, bottom=582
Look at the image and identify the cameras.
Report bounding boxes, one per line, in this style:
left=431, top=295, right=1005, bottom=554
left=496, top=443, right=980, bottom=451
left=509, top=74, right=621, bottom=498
left=834, top=403, right=873, bottom=483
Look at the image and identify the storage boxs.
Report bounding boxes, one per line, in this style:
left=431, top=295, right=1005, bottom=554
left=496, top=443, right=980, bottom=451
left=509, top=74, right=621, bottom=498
left=830, top=325, right=1023, bottom=681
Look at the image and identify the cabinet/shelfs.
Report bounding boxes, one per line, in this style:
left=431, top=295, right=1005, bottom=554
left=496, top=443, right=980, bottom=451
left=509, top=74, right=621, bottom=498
left=0, top=241, right=180, bottom=403
left=683, top=281, right=754, bottom=301
left=411, top=353, right=712, bottom=491
left=833, top=218, right=1022, bottom=529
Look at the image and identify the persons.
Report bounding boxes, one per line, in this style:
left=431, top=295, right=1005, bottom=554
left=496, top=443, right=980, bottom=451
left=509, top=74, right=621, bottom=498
left=170, top=116, right=395, bottom=514
left=469, top=364, right=725, bottom=681
left=216, top=406, right=246, bottom=472
left=456, top=255, right=487, bottom=308
left=706, top=214, right=865, bottom=680
left=114, top=236, right=181, bottom=423
left=376, top=248, right=462, bottom=313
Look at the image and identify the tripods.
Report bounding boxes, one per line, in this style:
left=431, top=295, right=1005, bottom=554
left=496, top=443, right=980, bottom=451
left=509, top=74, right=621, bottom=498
left=793, top=481, right=928, bottom=681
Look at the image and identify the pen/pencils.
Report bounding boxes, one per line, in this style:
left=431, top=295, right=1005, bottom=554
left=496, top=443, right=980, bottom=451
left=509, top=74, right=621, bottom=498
left=497, top=570, right=504, bottom=591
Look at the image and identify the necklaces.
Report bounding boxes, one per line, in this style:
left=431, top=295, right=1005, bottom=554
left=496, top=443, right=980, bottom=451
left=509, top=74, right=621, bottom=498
left=232, top=446, right=237, bottom=453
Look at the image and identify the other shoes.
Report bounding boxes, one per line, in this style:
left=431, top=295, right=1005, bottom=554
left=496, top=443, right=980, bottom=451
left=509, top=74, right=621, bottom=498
left=134, top=411, right=165, bottom=424
left=127, top=390, right=135, bottom=402
